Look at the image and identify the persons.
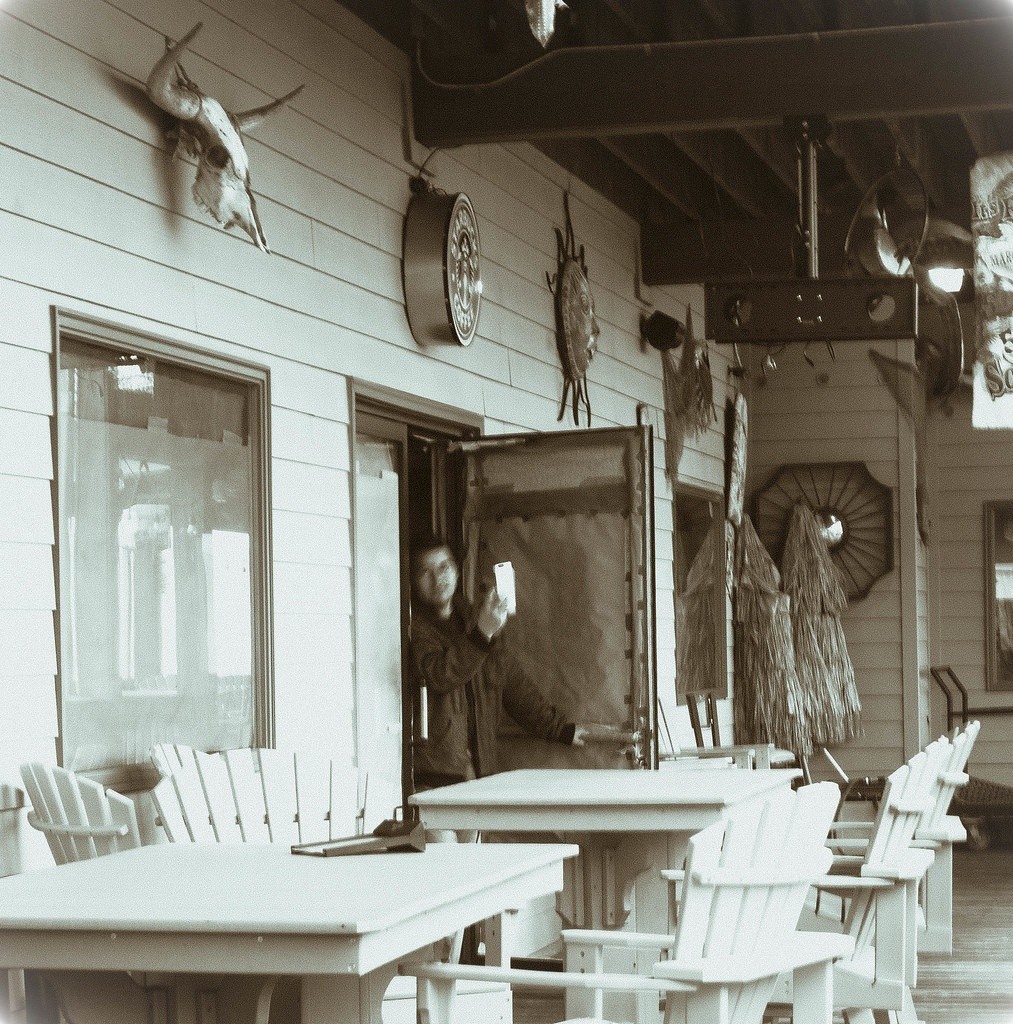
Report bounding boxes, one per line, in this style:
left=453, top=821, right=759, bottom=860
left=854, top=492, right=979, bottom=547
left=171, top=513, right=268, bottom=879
left=409, top=534, right=589, bottom=961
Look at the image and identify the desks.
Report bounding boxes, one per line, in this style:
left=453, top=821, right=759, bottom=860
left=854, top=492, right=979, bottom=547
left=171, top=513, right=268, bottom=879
left=0, top=844, right=579, bottom=1024
left=407, top=767, right=804, bottom=970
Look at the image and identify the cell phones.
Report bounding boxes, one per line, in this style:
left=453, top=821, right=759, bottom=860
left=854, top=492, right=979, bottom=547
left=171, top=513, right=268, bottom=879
left=494, top=562, right=518, bottom=617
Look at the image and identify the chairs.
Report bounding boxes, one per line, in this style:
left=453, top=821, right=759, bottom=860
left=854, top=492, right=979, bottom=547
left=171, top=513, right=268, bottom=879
left=19, top=743, right=369, bottom=1024
left=397, top=718, right=979, bottom=1024
